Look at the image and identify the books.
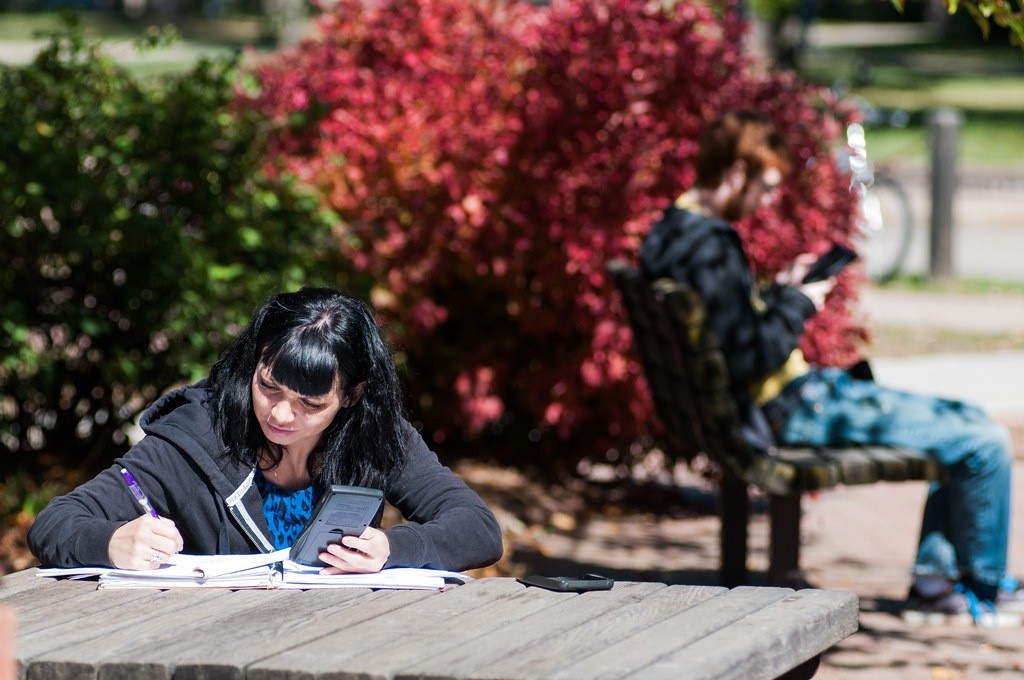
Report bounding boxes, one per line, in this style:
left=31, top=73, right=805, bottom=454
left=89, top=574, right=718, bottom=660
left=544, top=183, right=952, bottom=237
left=37, top=547, right=463, bottom=591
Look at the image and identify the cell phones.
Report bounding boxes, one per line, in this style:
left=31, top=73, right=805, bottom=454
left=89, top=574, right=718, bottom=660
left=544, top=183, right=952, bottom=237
left=800, top=244, right=856, bottom=284
left=516, top=572, right=613, bottom=592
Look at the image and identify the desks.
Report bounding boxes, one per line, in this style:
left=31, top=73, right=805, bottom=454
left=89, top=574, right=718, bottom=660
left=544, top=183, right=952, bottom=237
left=0, top=563, right=861, bottom=678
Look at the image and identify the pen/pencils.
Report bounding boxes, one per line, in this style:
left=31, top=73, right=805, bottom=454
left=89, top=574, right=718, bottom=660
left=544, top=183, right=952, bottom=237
left=120, top=468, right=179, bottom=554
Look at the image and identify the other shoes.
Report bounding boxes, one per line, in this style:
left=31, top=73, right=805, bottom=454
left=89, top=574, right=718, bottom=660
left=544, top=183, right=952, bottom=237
left=900, top=579, right=1024, bottom=629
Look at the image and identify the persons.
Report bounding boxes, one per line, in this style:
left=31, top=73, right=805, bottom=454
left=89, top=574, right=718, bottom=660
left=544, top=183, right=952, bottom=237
left=26, top=285, right=504, bottom=575
left=638, top=108, right=1024, bottom=630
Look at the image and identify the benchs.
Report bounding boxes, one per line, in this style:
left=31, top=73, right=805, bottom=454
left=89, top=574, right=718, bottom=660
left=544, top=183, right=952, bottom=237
left=607, top=261, right=966, bottom=577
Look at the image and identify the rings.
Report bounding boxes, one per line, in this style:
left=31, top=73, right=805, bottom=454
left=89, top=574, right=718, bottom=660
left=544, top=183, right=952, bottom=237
left=151, top=551, right=162, bottom=563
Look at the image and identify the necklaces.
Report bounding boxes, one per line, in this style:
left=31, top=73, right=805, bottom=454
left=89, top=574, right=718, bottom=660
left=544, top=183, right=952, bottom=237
left=268, top=455, right=309, bottom=487
